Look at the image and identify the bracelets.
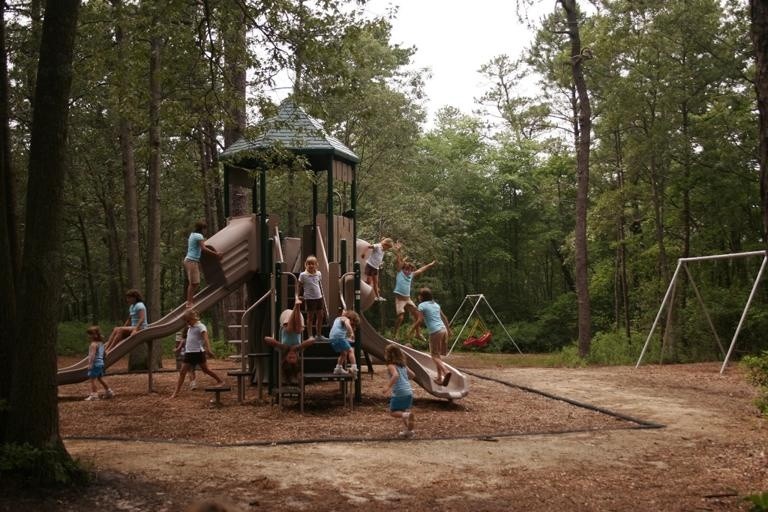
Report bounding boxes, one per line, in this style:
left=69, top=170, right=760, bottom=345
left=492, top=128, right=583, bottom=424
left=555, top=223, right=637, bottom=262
left=296, top=345, right=302, bottom=351
left=270, top=339, right=276, bottom=345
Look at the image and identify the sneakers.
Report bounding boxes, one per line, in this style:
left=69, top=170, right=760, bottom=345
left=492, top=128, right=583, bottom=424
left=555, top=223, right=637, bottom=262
left=85, top=395, right=100, bottom=401
left=406, top=414, right=414, bottom=431
left=415, top=335, right=427, bottom=341
left=443, top=371, right=452, bottom=387
left=433, top=376, right=442, bottom=385
left=316, top=335, right=329, bottom=341
left=398, top=429, right=414, bottom=436
left=103, top=391, right=115, bottom=398
left=374, top=296, right=386, bottom=302
left=334, top=368, right=358, bottom=380
left=190, top=381, right=196, bottom=390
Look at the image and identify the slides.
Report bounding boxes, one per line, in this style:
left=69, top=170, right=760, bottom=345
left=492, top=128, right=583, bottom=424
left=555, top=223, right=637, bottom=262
left=56, top=214, right=255, bottom=383
left=340, top=272, right=469, bottom=399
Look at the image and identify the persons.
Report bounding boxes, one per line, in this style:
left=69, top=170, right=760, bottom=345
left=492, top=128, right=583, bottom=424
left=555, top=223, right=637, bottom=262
left=297, top=255, right=330, bottom=342
left=364, top=237, right=394, bottom=301
left=264, top=298, right=315, bottom=379
left=104, top=289, right=147, bottom=354
left=382, top=344, right=415, bottom=437
left=183, top=222, right=225, bottom=308
left=405, top=288, right=454, bottom=387
left=168, top=309, right=226, bottom=400
left=328, top=310, right=361, bottom=381
left=170, top=326, right=197, bottom=391
left=392, top=253, right=436, bottom=343
left=83, top=325, right=115, bottom=402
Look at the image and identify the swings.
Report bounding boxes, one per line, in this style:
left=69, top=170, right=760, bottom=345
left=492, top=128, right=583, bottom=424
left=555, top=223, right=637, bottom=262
left=463, top=295, right=491, bottom=349
